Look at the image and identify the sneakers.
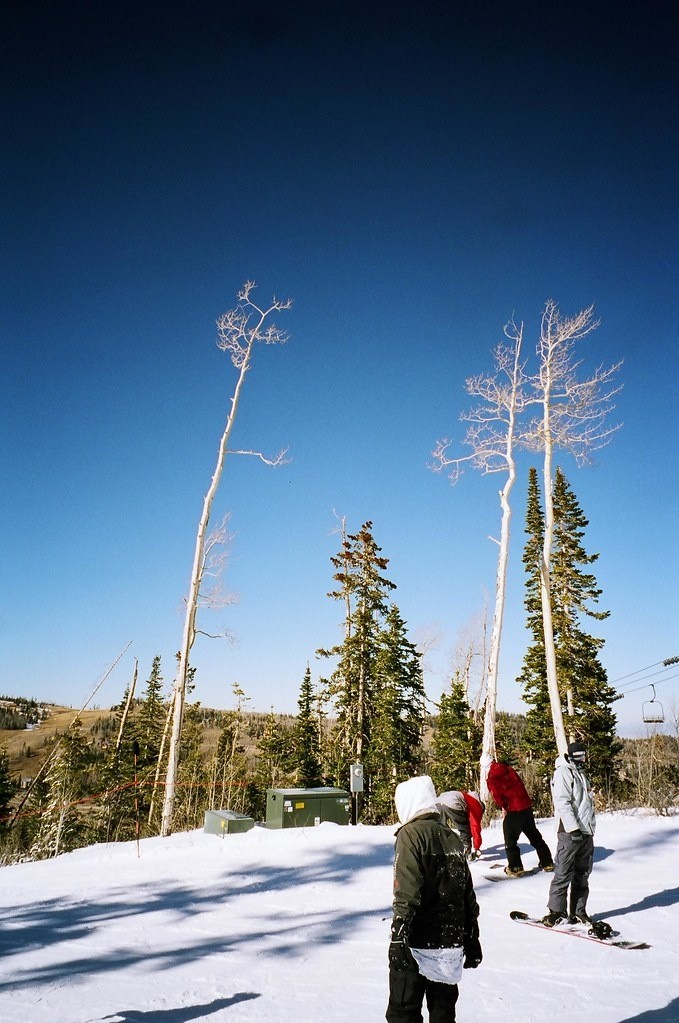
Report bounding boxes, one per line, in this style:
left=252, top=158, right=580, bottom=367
left=538, top=863, right=556, bottom=872
left=544, top=911, right=592, bottom=928
left=504, top=867, right=524, bottom=877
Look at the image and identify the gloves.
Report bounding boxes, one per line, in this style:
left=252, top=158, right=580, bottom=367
left=388, top=919, right=410, bottom=973
left=570, top=829, right=584, bottom=844
left=463, top=938, right=484, bottom=969
left=471, top=849, right=482, bottom=863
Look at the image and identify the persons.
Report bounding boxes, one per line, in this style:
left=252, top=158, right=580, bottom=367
left=543, top=742, right=596, bottom=927
left=480, top=752, right=555, bottom=877
left=437, top=791, right=485, bottom=861
left=385, top=775, right=482, bottom=1023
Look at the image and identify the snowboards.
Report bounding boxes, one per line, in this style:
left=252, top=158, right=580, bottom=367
left=481, top=863, right=555, bottom=882
left=509, top=911, right=648, bottom=949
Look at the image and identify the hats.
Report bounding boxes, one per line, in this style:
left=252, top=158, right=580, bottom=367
left=568, top=743, right=585, bottom=760
